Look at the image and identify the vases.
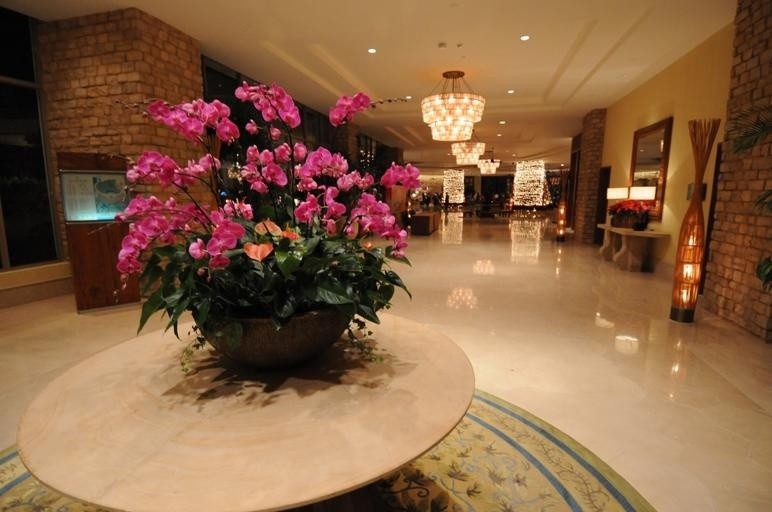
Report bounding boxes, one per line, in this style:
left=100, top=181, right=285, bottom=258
left=188, top=305, right=353, bottom=370
left=615, top=214, right=640, bottom=227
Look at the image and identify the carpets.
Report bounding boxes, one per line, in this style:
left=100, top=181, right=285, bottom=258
left=1, top=379, right=658, bottom=512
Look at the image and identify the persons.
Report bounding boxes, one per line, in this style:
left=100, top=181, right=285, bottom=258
left=422, top=186, right=481, bottom=218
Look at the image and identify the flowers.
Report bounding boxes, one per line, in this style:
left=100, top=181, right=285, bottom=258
left=608, top=199, right=651, bottom=231
left=86, top=79, right=422, bottom=373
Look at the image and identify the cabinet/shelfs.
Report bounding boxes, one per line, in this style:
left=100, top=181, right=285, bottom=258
left=57, top=151, right=140, bottom=313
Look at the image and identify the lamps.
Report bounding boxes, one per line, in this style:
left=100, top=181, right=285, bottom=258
left=670, top=117, right=721, bottom=324
left=477, top=151, right=500, bottom=175
left=451, top=129, right=486, bottom=166
left=420, top=71, right=485, bottom=142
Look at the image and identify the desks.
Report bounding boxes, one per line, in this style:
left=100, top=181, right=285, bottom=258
left=17, top=321, right=476, bottom=512
left=412, top=210, right=441, bottom=235
left=598, top=224, right=670, bottom=272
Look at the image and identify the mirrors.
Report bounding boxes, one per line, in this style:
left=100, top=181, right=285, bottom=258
left=628, top=116, right=673, bottom=224
